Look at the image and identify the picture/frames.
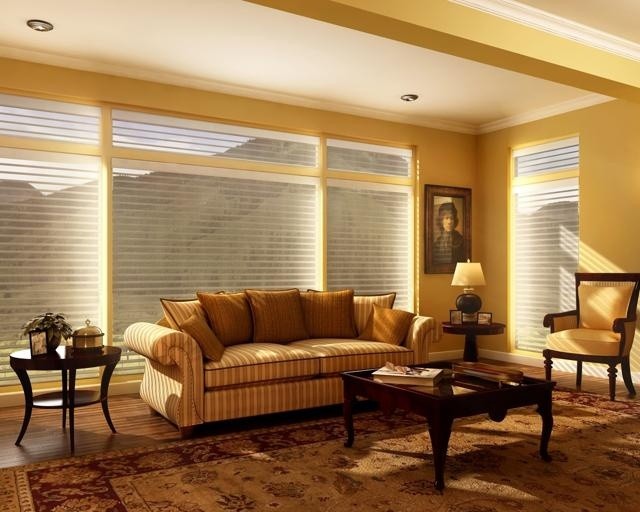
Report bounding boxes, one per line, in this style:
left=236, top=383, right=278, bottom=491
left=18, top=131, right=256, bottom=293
left=423, top=184, right=472, bottom=274
left=477, top=312, right=492, bottom=325
left=449, top=309, right=463, bottom=324
left=29, top=329, right=49, bottom=356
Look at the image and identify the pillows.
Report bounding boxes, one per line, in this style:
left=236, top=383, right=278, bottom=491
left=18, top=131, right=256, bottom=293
left=158, top=291, right=227, bottom=331
left=300, top=289, right=359, bottom=338
left=195, top=291, right=253, bottom=346
left=356, top=303, right=416, bottom=346
left=180, top=310, right=223, bottom=361
left=306, top=288, right=396, bottom=337
left=244, top=288, right=310, bottom=343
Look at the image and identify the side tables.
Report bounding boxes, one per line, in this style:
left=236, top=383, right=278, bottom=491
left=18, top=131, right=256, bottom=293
left=8, top=346, right=121, bottom=458
left=442, top=322, right=506, bottom=362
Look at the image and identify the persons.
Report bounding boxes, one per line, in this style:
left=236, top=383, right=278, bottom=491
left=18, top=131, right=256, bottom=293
left=433, top=202, right=464, bottom=265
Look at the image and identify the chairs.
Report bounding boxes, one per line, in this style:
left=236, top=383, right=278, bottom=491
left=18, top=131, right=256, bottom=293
left=543, top=273, right=640, bottom=402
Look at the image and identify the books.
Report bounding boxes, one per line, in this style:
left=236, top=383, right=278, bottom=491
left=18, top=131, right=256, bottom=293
left=371, top=365, right=444, bottom=387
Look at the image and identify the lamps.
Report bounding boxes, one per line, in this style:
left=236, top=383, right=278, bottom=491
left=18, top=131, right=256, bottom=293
left=450, top=259, right=486, bottom=322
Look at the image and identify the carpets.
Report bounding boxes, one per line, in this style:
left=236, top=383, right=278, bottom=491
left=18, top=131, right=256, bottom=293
left=0, top=385, right=640, bottom=512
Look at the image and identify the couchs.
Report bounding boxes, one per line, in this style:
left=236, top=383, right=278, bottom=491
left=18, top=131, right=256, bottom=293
left=123, top=314, right=443, bottom=441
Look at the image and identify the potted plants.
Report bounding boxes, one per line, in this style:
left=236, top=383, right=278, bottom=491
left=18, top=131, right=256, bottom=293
left=18, top=311, right=72, bottom=351
